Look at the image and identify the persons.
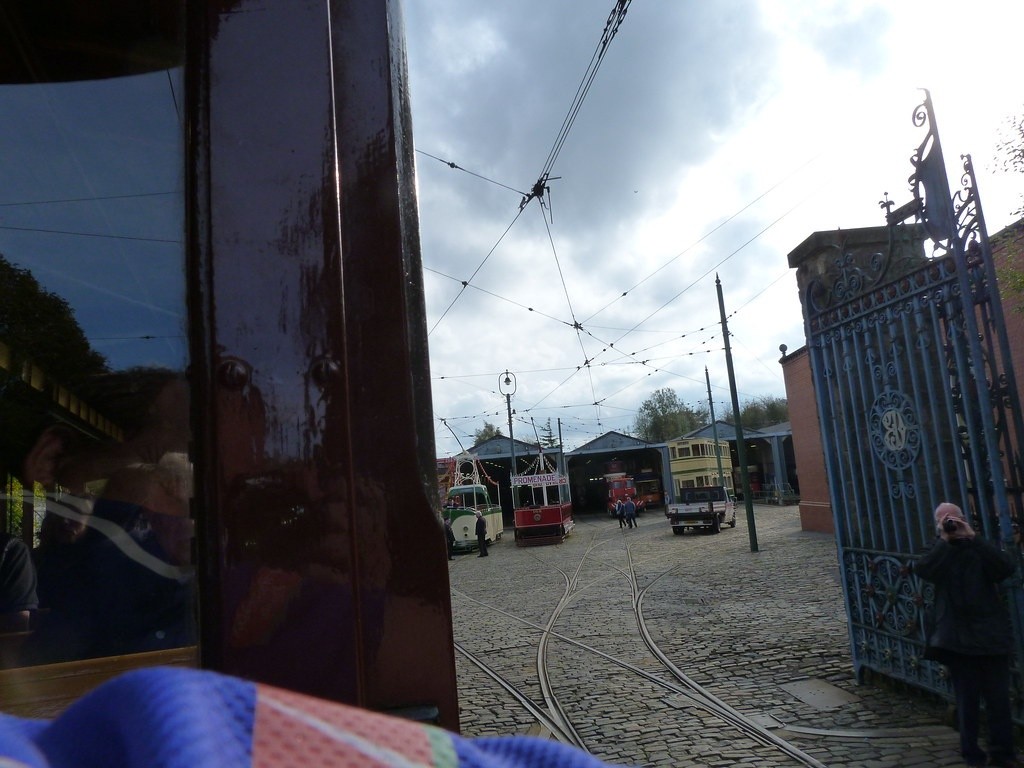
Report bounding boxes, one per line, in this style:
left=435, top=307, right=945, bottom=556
left=0, top=367, right=388, bottom=711
left=913, top=502, right=1024, bottom=768
left=624, top=497, right=637, bottom=528
left=475, top=511, right=488, bottom=557
left=616, top=499, right=626, bottom=528
left=444, top=517, right=455, bottom=560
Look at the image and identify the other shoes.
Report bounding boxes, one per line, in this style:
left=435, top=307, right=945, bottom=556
left=477, top=554, right=488, bottom=557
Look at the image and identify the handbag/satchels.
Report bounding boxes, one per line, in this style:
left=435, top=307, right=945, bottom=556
left=617, top=511, right=620, bottom=514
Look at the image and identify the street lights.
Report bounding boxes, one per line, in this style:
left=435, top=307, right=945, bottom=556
left=499, top=369, right=521, bottom=509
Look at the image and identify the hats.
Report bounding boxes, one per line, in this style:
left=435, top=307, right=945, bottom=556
left=617, top=500, right=621, bottom=503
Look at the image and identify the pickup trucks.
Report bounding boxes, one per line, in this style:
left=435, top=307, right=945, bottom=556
left=664, top=486, right=737, bottom=535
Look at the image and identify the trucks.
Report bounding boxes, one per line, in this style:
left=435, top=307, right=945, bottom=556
left=635, top=473, right=663, bottom=509
